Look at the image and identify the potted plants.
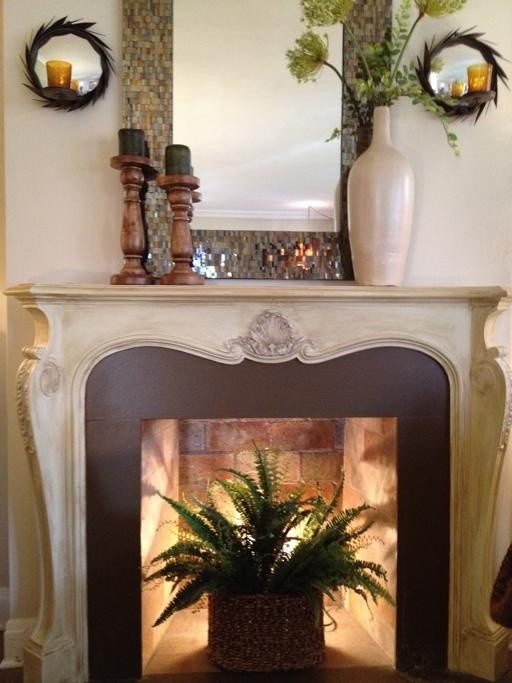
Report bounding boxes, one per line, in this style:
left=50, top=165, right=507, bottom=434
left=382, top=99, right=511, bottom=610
left=135, top=442, right=392, bottom=676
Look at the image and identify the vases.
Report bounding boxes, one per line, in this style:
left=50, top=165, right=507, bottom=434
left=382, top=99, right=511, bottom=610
left=345, top=104, right=417, bottom=286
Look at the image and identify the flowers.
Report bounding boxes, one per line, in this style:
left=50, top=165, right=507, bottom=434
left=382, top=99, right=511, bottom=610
left=286, top=0, right=477, bottom=160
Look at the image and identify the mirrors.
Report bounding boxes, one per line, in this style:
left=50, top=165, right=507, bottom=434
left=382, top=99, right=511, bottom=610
left=18, top=11, right=117, bottom=110
left=119, top=0, right=396, bottom=288
left=414, top=22, right=505, bottom=125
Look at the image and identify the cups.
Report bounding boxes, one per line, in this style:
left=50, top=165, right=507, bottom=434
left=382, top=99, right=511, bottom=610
left=46, top=59, right=73, bottom=86
left=467, top=62, right=493, bottom=91
left=449, top=79, right=466, bottom=97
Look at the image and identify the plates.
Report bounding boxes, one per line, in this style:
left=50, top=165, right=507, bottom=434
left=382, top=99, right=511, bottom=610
left=42, top=86, right=76, bottom=97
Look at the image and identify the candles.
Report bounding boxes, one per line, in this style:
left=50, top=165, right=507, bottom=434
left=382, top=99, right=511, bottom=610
left=119, top=125, right=147, bottom=156
left=164, top=144, right=191, bottom=176
